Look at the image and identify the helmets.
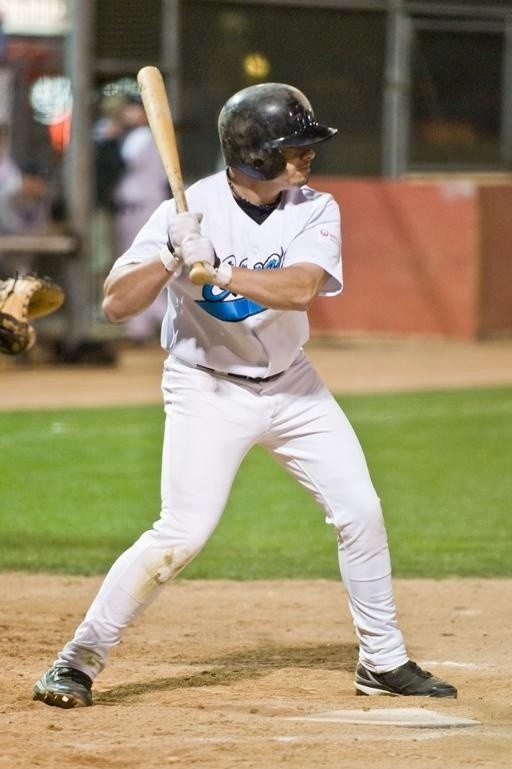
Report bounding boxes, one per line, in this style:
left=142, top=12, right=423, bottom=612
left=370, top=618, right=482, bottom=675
left=214, top=80, right=342, bottom=183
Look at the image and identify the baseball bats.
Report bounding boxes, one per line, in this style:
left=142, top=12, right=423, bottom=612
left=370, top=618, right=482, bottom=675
left=137, top=65, right=208, bottom=285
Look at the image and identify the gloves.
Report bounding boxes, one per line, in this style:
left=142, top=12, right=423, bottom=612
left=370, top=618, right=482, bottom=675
left=156, top=211, right=205, bottom=276
left=173, top=237, right=234, bottom=292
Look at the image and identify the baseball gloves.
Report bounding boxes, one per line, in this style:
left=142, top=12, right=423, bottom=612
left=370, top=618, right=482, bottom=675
left=1, top=270, right=65, bottom=354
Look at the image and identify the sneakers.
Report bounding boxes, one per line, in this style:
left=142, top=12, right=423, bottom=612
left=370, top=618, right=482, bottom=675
left=31, top=662, right=95, bottom=712
left=350, top=658, right=459, bottom=700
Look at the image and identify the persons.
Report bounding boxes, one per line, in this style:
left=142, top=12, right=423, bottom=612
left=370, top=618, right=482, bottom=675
left=1, top=74, right=168, bottom=366
left=30, top=81, right=460, bottom=708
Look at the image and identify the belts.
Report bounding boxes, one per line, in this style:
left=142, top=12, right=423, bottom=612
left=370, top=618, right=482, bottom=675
left=175, top=357, right=286, bottom=384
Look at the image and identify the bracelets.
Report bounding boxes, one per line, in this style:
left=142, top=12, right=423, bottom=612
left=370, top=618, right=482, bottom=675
left=215, top=260, right=235, bottom=289
left=162, top=243, right=180, bottom=274
